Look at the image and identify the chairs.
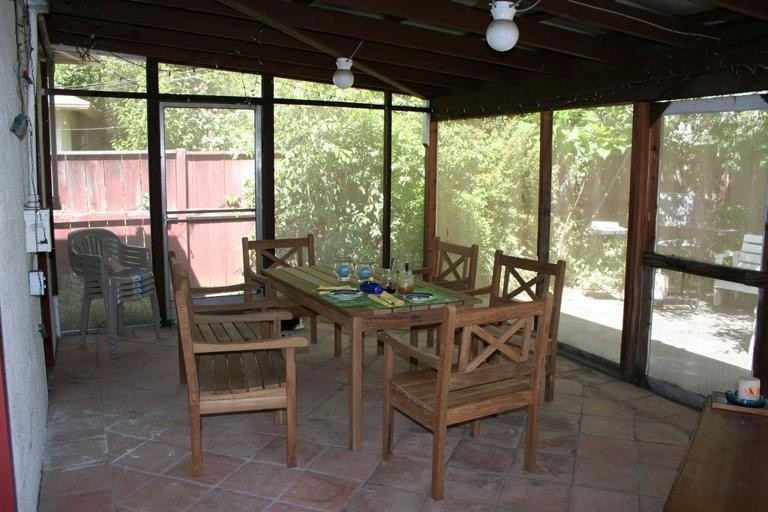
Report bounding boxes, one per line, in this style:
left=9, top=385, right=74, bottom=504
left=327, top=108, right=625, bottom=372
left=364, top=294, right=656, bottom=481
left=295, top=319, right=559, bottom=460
left=66, top=227, right=165, bottom=358
left=240, top=232, right=342, bottom=359
left=402, top=237, right=481, bottom=365
left=167, top=240, right=279, bottom=382
left=375, top=291, right=556, bottom=503
left=173, top=275, right=311, bottom=482
left=446, top=248, right=564, bottom=403
left=713, top=233, right=763, bottom=306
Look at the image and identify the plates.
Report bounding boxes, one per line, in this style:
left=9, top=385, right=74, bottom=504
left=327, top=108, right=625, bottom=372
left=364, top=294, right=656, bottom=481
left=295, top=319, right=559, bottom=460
left=725, top=391, right=765, bottom=408
left=326, top=289, right=362, bottom=301
left=401, top=293, right=435, bottom=303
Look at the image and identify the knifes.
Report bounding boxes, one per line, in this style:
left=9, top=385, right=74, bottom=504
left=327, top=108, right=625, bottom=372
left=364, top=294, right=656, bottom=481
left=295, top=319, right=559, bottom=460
left=316, top=288, right=359, bottom=292
left=377, top=295, right=393, bottom=305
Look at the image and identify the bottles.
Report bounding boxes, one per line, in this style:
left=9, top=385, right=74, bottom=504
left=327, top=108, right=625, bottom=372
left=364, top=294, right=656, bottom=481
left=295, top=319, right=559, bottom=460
left=359, top=280, right=382, bottom=295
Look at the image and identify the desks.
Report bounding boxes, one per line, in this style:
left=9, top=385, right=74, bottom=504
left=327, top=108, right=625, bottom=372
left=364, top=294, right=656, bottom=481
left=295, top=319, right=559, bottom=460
left=661, top=389, right=765, bottom=511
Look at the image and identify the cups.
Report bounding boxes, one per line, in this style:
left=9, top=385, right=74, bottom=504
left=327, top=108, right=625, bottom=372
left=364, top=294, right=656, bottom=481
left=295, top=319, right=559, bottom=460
left=396, top=271, right=414, bottom=295
left=354, top=260, right=377, bottom=283
left=735, top=374, right=760, bottom=403
left=332, top=257, right=354, bottom=282
left=375, top=267, right=399, bottom=293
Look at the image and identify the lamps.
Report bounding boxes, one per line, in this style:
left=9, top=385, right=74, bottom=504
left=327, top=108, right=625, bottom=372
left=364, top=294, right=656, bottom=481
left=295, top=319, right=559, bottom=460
left=327, top=39, right=365, bottom=91
left=481, top=0, right=545, bottom=56
